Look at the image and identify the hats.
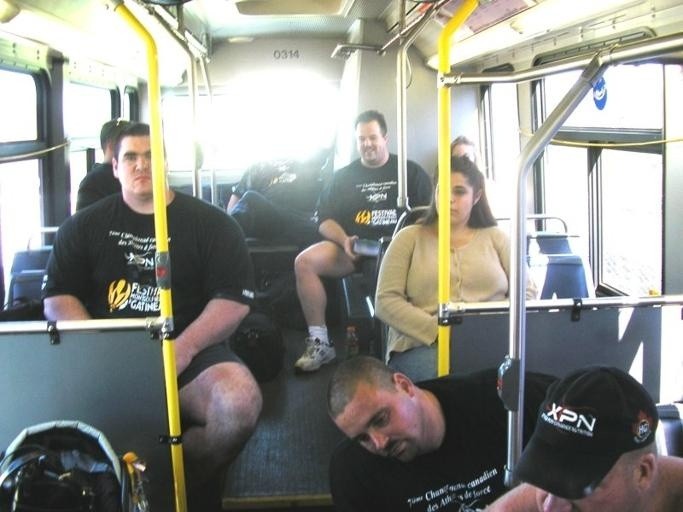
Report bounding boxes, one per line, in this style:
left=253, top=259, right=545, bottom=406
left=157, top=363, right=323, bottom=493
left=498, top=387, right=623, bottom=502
left=516, top=366, right=658, bottom=499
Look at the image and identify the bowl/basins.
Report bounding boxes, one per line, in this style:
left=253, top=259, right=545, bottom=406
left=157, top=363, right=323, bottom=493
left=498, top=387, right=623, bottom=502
left=352, top=238, right=381, bottom=256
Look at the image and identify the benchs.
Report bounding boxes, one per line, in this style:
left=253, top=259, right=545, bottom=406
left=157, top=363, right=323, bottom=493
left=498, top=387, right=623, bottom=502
left=5, top=181, right=596, bottom=310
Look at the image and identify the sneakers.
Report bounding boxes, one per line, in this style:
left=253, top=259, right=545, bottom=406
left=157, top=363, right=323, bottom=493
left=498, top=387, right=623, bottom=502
left=294, top=335, right=337, bottom=371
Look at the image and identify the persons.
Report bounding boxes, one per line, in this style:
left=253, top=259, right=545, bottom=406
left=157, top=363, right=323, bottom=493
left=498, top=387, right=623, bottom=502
left=481, top=366, right=683, bottom=511
left=448, top=135, right=478, bottom=170
left=294, top=109, right=433, bottom=372
left=37, top=120, right=264, bottom=512
left=373, top=154, right=539, bottom=362
left=325, top=355, right=565, bottom=512
left=225, top=132, right=335, bottom=248
